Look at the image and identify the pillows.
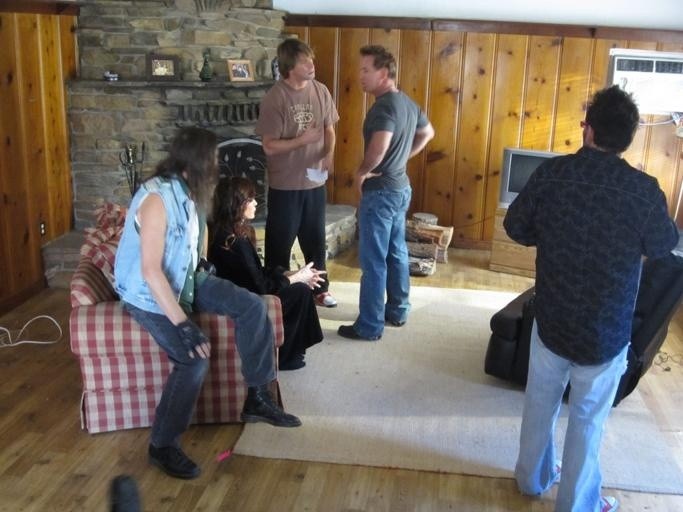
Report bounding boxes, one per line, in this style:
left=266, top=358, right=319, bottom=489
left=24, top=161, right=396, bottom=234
left=79, top=198, right=129, bottom=293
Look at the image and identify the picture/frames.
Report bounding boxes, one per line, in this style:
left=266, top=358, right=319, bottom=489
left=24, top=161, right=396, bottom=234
left=146, top=54, right=182, bottom=81
left=226, top=59, right=254, bottom=82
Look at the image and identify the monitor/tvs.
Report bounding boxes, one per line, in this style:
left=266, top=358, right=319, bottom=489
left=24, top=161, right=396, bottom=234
left=498, top=148, right=558, bottom=208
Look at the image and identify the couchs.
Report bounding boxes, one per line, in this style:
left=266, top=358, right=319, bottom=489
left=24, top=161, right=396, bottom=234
left=485, top=228, right=683, bottom=406
left=69, top=255, right=284, bottom=434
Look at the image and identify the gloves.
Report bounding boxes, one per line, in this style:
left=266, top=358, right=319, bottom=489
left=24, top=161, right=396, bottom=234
left=177, top=318, right=210, bottom=351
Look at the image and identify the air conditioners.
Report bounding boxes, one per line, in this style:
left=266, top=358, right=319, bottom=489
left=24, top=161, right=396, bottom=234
left=607, top=48, right=683, bottom=115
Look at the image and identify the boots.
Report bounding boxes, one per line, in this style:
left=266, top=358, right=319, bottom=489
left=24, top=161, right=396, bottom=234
left=240, top=384, right=302, bottom=427
left=149, top=443, right=200, bottom=479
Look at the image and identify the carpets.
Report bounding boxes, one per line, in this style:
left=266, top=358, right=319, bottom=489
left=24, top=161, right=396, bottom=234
left=232, top=280, right=682, bottom=495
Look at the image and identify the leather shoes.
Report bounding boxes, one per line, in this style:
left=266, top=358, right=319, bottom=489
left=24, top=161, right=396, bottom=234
left=339, top=325, right=382, bottom=340
left=282, top=358, right=305, bottom=370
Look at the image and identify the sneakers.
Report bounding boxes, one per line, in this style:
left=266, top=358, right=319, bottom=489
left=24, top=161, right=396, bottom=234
left=312, top=292, right=337, bottom=308
left=599, top=496, right=620, bottom=512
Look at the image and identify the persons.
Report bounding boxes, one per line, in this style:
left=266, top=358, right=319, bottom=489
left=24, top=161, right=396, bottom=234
left=232, top=63, right=250, bottom=78
left=337, top=47, right=437, bottom=342
left=209, top=178, right=328, bottom=370
left=502, top=86, right=678, bottom=512
left=252, top=40, right=340, bottom=308
left=114, top=128, right=301, bottom=479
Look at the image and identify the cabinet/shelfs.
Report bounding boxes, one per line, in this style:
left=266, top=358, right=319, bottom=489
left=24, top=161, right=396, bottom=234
left=488, top=207, right=537, bottom=279
